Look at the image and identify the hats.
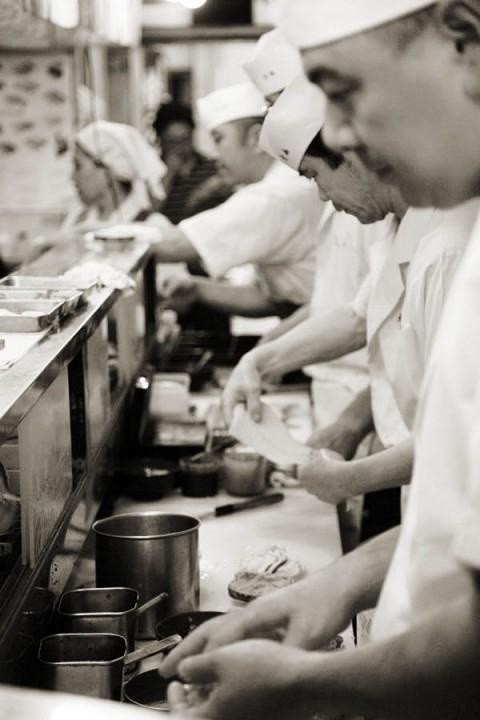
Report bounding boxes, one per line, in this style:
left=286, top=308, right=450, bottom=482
left=78, top=120, right=167, bottom=181
left=193, top=24, right=333, bottom=170
left=276, top=1, right=449, bottom=49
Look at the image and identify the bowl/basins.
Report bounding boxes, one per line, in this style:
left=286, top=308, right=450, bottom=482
left=119, top=457, right=179, bottom=501
left=156, top=610, right=229, bottom=657
left=124, top=667, right=178, bottom=715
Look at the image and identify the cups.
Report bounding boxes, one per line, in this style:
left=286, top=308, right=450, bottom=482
left=223, top=445, right=267, bottom=497
left=179, top=457, right=220, bottom=498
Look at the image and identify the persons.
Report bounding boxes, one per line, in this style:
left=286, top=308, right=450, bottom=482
left=60, top=120, right=188, bottom=276
left=127, top=2, right=480, bottom=720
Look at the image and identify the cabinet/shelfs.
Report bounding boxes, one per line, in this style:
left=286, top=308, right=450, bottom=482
left=0, top=227, right=158, bottom=632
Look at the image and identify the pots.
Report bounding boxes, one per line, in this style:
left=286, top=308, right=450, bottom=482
left=90, top=511, right=202, bottom=643
